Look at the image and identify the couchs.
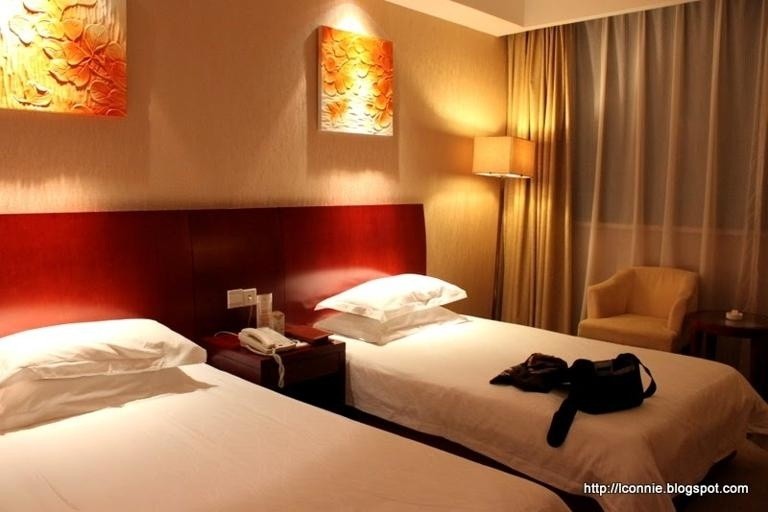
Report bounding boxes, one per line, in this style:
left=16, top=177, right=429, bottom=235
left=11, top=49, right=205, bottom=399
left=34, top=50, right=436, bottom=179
left=576, top=265, right=700, bottom=354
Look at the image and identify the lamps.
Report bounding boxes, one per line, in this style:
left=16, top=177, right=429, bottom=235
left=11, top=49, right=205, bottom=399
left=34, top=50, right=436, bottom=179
left=471, top=136, right=537, bottom=320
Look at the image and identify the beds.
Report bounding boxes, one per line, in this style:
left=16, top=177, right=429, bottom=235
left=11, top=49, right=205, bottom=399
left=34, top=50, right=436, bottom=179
left=312, top=272, right=768, bottom=511
left=2, top=317, right=575, bottom=511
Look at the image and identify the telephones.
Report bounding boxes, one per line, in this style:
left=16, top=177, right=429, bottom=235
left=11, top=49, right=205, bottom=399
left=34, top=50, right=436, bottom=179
left=238, top=326, right=296, bottom=358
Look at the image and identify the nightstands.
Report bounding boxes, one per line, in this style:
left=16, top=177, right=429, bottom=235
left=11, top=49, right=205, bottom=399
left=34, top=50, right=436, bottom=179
left=197, top=325, right=346, bottom=417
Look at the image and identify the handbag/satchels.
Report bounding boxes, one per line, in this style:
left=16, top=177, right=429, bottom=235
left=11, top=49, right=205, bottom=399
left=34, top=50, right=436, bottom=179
left=546, top=353, right=655, bottom=447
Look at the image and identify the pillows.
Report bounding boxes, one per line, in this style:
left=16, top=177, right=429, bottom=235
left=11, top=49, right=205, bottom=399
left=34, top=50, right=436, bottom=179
left=2, top=316, right=208, bottom=386
left=308, top=313, right=464, bottom=346
left=2, top=351, right=194, bottom=432
left=314, top=274, right=469, bottom=321
